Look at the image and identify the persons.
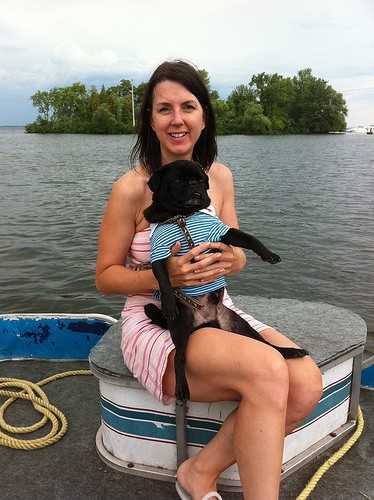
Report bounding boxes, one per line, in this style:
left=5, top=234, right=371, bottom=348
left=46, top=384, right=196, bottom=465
left=95, top=60, right=323, bottom=500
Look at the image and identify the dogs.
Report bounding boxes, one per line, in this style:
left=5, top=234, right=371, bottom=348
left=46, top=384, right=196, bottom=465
left=143, top=161, right=309, bottom=408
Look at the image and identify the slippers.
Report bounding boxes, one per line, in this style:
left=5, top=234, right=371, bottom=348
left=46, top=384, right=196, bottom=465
left=175, top=480, right=223, bottom=500
